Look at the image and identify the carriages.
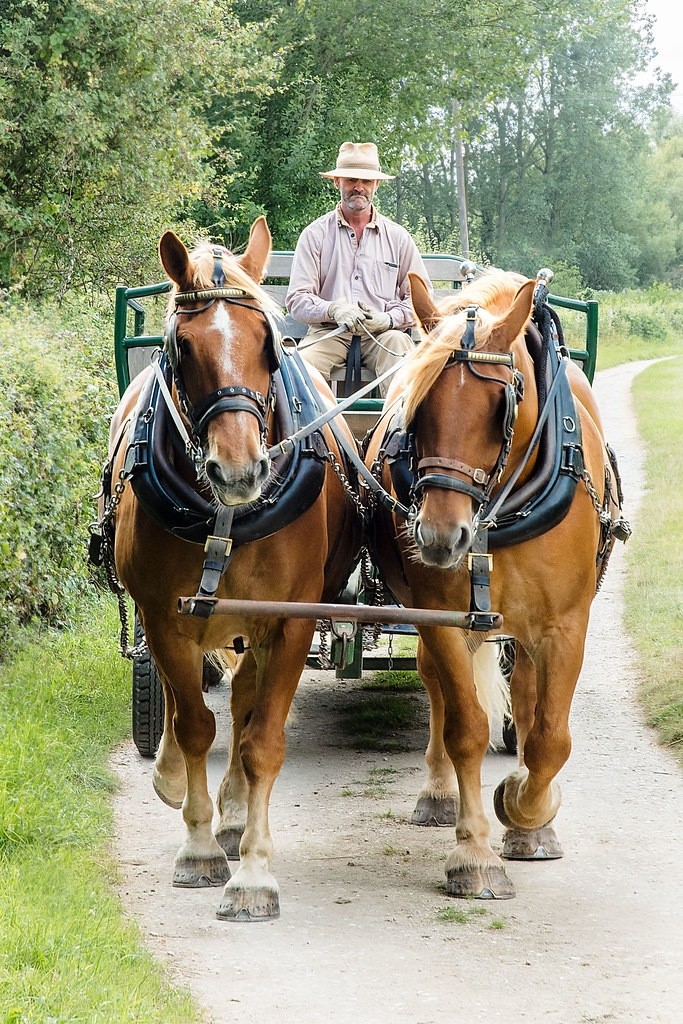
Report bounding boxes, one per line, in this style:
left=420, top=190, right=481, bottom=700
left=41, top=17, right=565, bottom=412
left=88, top=214, right=634, bottom=924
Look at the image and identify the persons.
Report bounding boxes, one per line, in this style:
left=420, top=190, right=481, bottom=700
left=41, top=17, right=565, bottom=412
left=283, top=138, right=434, bottom=398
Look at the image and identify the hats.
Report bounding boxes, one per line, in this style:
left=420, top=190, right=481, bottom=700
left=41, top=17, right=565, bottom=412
left=318, top=141, right=396, bottom=180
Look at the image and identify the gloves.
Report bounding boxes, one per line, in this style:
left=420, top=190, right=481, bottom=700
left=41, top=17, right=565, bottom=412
left=353, top=299, right=391, bottom=336
left=327, top=298, right=366, bottom=334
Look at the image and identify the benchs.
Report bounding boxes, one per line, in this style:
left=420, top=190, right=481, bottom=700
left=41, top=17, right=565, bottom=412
left=259, top=250, right=472, bottom=408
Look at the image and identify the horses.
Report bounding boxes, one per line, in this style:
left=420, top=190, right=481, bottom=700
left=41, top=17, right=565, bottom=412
left=105, top=215, right=365, bottom=919
left=357, top=270, right=623, bottom=900
